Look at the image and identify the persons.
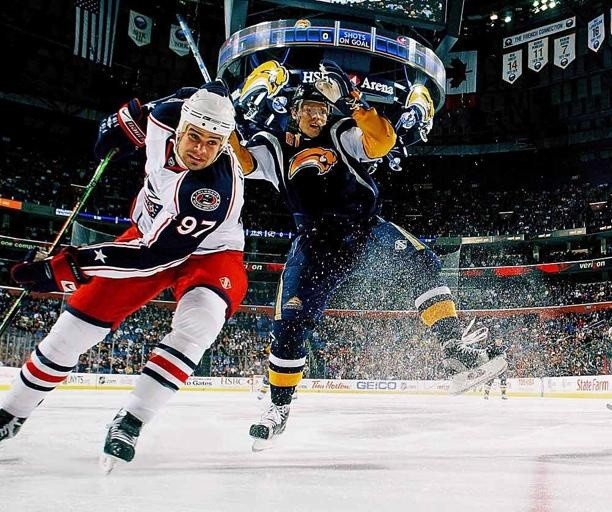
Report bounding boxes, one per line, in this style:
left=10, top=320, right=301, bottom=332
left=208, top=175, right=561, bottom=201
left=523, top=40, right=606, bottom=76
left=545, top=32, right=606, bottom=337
left=1, top=123, right=612, bottom=399
left=210, top=58, right=494, bottom=440
left=0, top=82, right=249, bottom=462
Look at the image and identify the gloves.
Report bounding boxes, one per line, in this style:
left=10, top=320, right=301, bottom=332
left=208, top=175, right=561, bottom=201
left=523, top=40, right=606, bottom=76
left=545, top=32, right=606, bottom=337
left=313, top=55, right=370, bottom=115
left=93, top=98, right=148, bottom=157
left=8, top=245, right=92, bottom=295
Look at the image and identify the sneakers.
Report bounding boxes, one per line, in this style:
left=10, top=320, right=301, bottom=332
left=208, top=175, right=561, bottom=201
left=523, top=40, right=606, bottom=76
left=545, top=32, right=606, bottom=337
left=245, top=401, right=291, bottom=441
left=103, top=408, right=144, bottom=465
left=440, top=335, right=507, bottom=377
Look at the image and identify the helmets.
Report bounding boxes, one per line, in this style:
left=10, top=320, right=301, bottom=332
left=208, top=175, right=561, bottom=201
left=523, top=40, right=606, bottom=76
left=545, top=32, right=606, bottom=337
left=290, top=80, right=330, bottom=113
left=173, top=88, right=237, bottom=170
